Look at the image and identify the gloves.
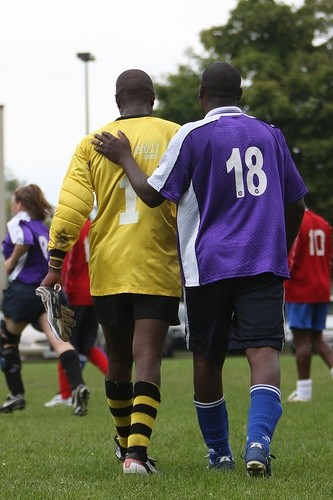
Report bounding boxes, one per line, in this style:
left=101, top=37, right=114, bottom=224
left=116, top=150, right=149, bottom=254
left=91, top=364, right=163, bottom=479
left=35, top=283, right=75, bottom=342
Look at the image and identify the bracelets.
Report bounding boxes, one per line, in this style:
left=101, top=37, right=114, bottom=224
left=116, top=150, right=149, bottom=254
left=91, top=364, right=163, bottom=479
left=47, top=260, right=63, bottom=269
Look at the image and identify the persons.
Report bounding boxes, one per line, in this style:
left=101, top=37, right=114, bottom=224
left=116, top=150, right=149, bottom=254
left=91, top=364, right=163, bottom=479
left=283, top=204, right=333, bottom=403
left=0, top=184, right=90, bottom=417
left=40, top=69, right=183, bottom=473
left=44, top=217, right=108, bottom=407
left=89, top=63, right=308, bottom=478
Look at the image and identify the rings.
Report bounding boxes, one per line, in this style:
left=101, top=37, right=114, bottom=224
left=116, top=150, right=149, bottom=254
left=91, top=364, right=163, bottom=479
left=100, top=142, right=103, bottom=146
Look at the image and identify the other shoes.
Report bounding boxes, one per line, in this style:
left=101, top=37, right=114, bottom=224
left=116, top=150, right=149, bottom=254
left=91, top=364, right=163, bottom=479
left=0, top=393, right=24, bottom=414
left=121, top=452, right=157, bottom=475
left=240, top=447, right=276, bottom=477
left=286, top=391, right=307, bottom=402
left=70, top=384, right=89, bottom=416
left=204, top=454, right=235, bottom=470
left=43, top=395, right=72, bottom=407
left=115, top=445, right=128, bottom=462
